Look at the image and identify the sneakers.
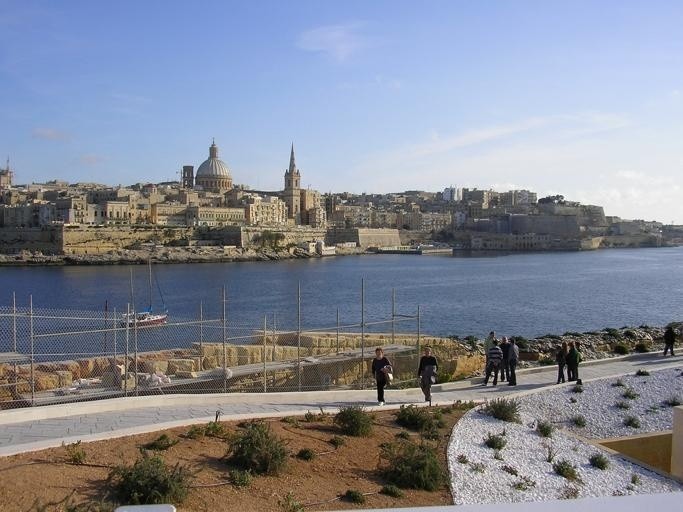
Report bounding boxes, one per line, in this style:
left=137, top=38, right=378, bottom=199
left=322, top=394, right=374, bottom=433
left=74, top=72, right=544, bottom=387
left=378, top=401, right=386, bottom=406
left=482, top=379, right=516, bottom=386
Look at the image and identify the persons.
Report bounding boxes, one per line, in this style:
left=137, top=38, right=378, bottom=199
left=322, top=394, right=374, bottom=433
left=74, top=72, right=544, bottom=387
left=568, top=341, right=577, bottom=380
left=480, top=339, right=503, bottom=386
left=662, top=325, right=677, bottom=356
left=418, top=346, right=438, bottom=403
left=482, top=330, right=502, bottom=366
left=498, top=335, right=510, bottom=381
left=575, top=341, right=581, bottom=378
left=555, top=342, right=568, bottom=383
left=369, top=346, right=392, bottom=406
left=508, top=336, right=518, bottom=385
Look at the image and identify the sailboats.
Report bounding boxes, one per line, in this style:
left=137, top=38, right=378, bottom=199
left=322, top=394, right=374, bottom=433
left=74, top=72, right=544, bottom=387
left=119, top=254, right=170, bottom=329
